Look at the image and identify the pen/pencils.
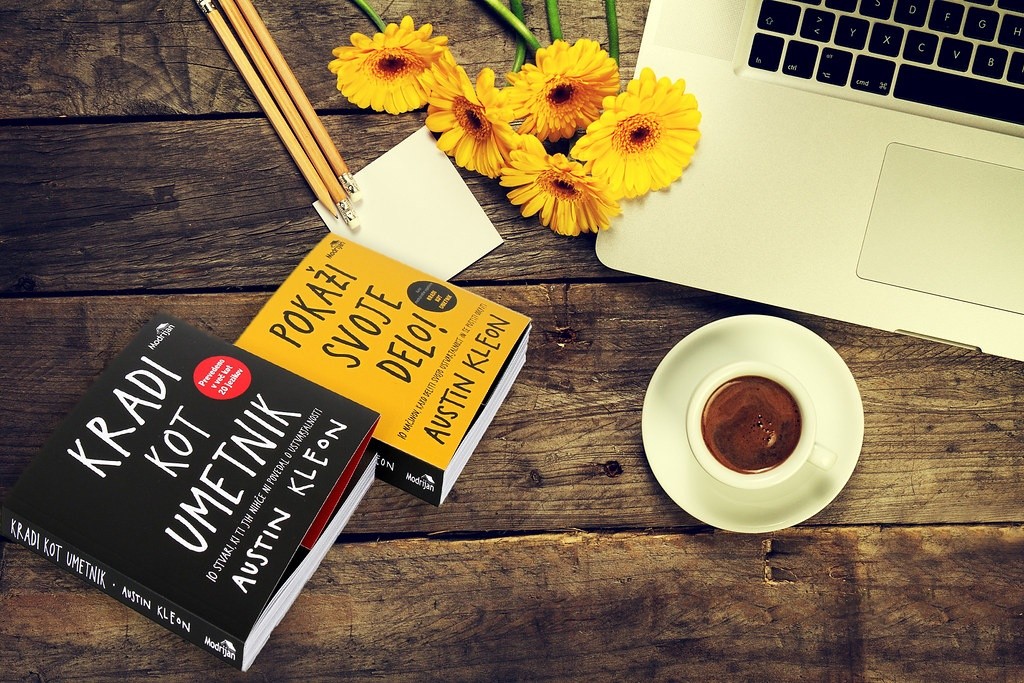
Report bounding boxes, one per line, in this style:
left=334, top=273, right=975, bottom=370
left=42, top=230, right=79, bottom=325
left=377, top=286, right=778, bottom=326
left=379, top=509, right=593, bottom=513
left=189, top=0, right=368, bottom=233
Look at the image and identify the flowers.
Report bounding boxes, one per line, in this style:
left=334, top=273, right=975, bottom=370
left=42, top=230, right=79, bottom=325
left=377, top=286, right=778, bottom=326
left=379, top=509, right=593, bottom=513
left=325, top=0, right=702, bottom=237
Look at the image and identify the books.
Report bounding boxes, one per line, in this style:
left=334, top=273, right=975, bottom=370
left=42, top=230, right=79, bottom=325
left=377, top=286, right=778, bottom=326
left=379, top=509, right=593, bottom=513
left=237, top=231, right=531, bottom=509
left=2, top=314, right=374, bottom=672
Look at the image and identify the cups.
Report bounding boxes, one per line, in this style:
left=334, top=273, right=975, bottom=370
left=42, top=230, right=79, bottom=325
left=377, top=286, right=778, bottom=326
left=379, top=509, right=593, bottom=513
left=687, top=356, right=818, bottom=493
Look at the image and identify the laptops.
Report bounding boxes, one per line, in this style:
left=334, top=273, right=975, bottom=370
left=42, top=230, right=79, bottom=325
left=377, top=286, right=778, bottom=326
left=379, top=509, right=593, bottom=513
left=594, top=0, right=1024, bottom=365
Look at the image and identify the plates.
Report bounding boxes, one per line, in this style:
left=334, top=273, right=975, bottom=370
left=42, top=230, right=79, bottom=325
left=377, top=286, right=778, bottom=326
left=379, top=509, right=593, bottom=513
left=641, top=315, right=864, bottom=533
